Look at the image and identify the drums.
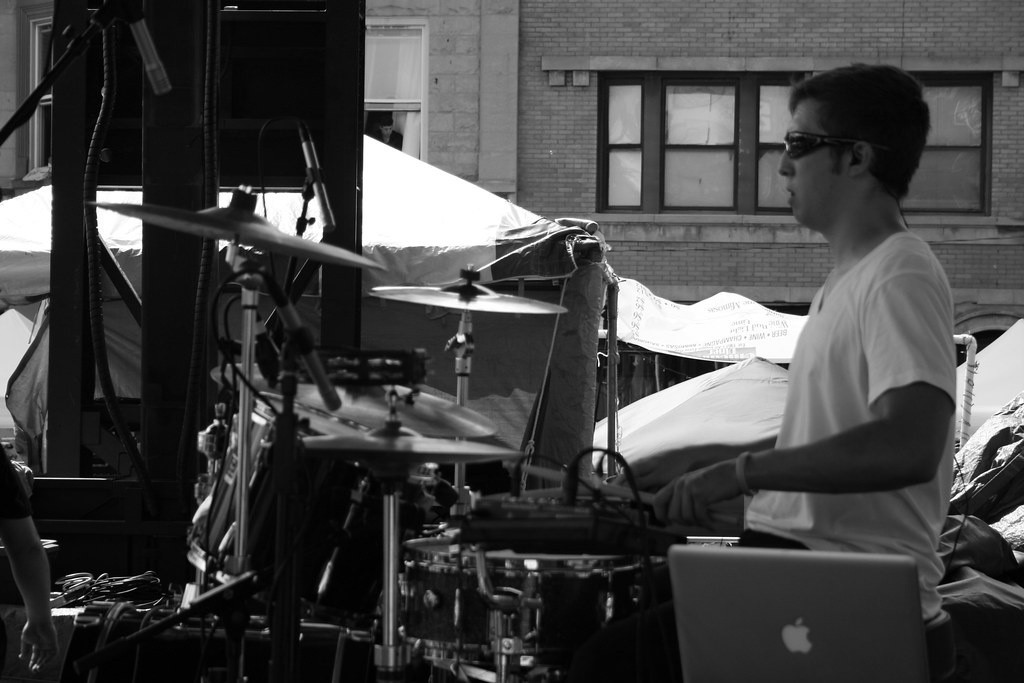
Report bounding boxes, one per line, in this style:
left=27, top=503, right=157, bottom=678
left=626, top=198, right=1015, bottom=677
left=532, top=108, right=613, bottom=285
left=182, top=395, right=447, bottom=638
left=403, top=539, right=638, bottom=653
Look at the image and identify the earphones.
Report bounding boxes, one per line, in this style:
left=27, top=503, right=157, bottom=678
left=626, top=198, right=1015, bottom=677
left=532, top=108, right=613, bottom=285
left=849, top=153, right=862, bottom=166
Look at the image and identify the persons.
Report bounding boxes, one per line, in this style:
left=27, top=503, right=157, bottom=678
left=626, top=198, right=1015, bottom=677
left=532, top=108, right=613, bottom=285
left=612, top=62, right=959, bottom=683
left=0, top=443, right=59, bottom=674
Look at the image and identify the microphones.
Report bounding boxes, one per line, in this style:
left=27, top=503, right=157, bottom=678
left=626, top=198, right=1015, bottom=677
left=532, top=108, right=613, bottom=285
left=299, top=121, right=337, bottom=233
left=263, top=271, right=341, bottom=414
left=109, top=0, right=173, bottom=96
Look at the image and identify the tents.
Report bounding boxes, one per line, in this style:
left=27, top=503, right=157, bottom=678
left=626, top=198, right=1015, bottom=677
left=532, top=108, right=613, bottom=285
left=955, top=318, right=1024, bottom=450
left=617, top=276, right=810, bottom=364
left=592, top=356, right=789, bottom=474
left=1, top=131, right=581, bottom=440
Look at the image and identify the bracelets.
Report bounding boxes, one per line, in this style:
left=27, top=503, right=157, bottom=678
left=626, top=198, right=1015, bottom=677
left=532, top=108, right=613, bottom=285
left=736, top=451, right=755, bottom=496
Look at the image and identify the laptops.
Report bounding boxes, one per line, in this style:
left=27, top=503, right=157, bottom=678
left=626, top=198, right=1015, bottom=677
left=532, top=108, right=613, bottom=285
left=668, top=546, right=934, bottom=683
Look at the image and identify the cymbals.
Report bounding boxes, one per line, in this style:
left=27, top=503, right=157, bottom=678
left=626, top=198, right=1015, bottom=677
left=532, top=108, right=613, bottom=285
left=301, top=437, right=520, bottom=463
left=286, top=376, right=497, bottom=437
left=90, top=199, right=385, bottom=271
left=373, top=280, right=568, bottom=314
left=208, top=361, right=315, bottom=396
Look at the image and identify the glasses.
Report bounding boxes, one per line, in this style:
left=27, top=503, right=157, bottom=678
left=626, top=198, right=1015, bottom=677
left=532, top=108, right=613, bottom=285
left=783, top=130, right=875, bottom=160
